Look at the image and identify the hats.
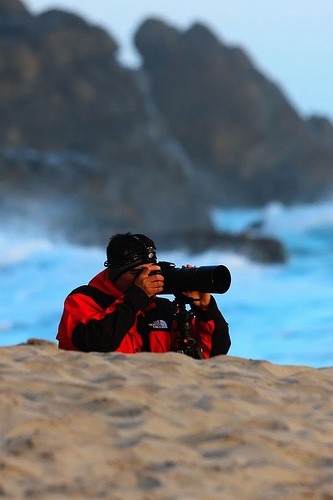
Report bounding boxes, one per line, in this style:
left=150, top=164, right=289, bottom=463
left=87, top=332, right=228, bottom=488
left=103, top=232, right=157, bottom=280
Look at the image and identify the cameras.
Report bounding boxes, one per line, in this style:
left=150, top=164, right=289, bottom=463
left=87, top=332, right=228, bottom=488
left=149, top=261, right=231, bottom=294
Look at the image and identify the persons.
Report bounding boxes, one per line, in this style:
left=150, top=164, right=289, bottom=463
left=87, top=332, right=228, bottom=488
left=55, top=232, right=231, bottom=360
